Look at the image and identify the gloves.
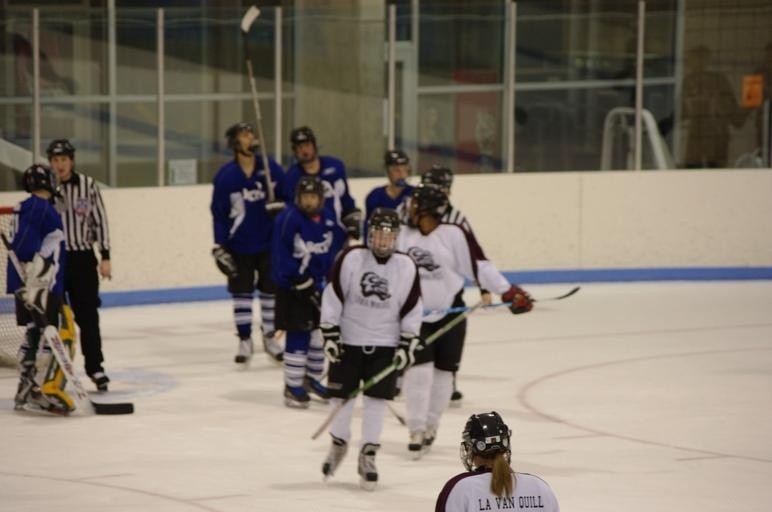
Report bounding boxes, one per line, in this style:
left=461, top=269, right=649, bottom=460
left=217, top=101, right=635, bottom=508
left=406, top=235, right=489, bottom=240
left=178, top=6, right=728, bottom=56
left=502, top=284, right=534, bottom=315
left=408, top=247, right=439, bottom=273
left=213, top=247, right=240, bottom=279
left=295, top=280, right=322, bottom=316
left=392, top=334, right=422, bottom=371
left=341, top=210, right=364, bottom=240
left=321, top=325, right=346, bottom=364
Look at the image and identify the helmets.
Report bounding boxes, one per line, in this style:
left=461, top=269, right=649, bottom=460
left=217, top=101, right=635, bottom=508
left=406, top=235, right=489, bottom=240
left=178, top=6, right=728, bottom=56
left=290, top=126, right=316, bottom=151
left=225, top=122, right=259, bottom=150
left=459, top=411, right=511, bottom=472
left=297, top=177, right=325, bottom=217
left=409, top=185, right=450, bottom=228
left=365, top=206, right=402, bottom=257
left=46, top=138, right=76, bottom=160
left=383, top=150, right=411, bottom=167
left=23, top=164, right=63, bottom=203
left=421, top=166, right=452, bottom=194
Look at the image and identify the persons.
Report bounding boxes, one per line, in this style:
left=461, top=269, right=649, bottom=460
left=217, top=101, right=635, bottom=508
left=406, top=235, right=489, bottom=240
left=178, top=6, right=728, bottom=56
left=738, top=41, right=772, bottom=167
left=38, top=138, right=113, bottom=387
left=6, top=161, right=81, bottom=416
left=433, top=411, right=562, bottom=511
left=682, top=46, right=738, bottom=167
left=512, top=105, right=534, bottom=172
left=613, top=40, right=681, bottom=169
left=207, top=123, right=537, bottom=484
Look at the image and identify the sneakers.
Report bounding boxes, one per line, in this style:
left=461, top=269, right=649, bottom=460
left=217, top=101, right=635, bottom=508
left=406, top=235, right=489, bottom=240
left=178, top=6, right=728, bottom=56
left=263, top=338, right=284, bottom=361
left=451, top=390, right=462, bottom=402
left=25, top=385, right=59, bottom=408
left=424, top=433, right=435, bottom=447
left=91, top=372, right=110, bottom=386
left=357, top=444, right=382, bottom=483
left=15, top=383, right=30, bottom=406
left=286, top=386, right=310, bottom=403
left=304, top=380, right=332, bottom=400
left=322, top=431, right=348, bottom=474
left=408, top=434, right=423, bottom=451
left=234, top=338, right=254, bottom=361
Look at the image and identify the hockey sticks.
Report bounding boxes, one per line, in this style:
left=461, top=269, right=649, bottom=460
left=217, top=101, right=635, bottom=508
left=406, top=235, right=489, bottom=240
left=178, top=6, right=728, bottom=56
left=312, top=295, right=485, bottom=441
left=384, top=400, right=407, bottom=428
left=239, top=5, right=274, bottom=201
left=423, top=286, right=581, bottom=314
left=1, top=231, right=132, bottom=415
